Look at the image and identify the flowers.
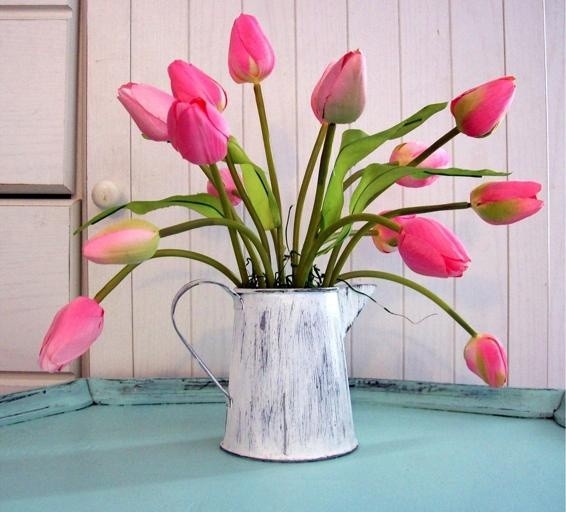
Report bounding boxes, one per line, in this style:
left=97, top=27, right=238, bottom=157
left=37, top=13, right=542, bottom=392
